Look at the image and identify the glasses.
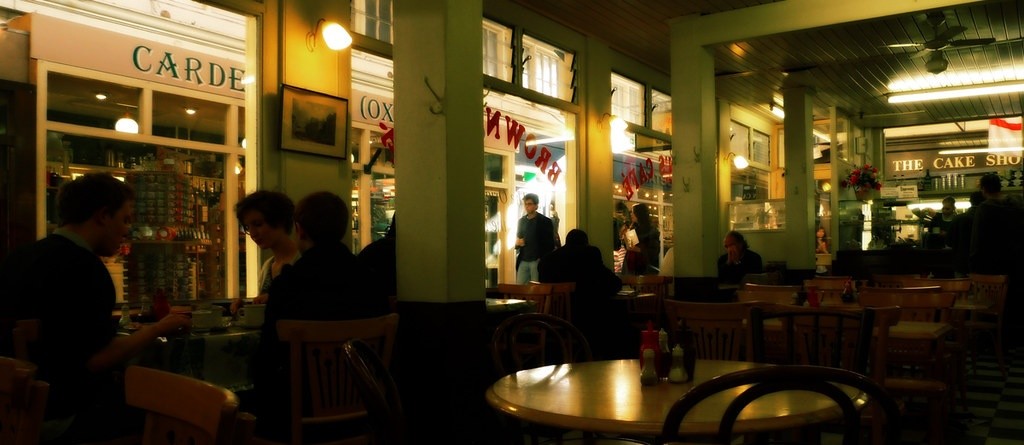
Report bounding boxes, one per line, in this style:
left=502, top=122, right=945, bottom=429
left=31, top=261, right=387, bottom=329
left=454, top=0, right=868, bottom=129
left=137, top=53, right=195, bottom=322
left=524, top=202, right=535, bottom=206
left=242, top=218, right=268, bottom=235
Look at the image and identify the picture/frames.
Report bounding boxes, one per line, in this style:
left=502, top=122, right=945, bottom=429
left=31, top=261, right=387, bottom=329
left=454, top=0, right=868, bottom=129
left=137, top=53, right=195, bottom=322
left=279, top=84, right=350, bottom=161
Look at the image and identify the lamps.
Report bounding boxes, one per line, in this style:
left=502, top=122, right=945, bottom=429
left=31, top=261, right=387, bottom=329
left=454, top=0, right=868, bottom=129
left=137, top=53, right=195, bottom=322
left=925, top=49, right=947, bottom=74
left=184, top=117, right=193, bottom=174
left=724, top=152, right=750, bottom=169
left=307, top=17, right=353, bottom=53
left=598, top=112, right=629, bottom=137
left=115, top=107, right=139, bottom=135
left=769, top=96, right=833, bottom=142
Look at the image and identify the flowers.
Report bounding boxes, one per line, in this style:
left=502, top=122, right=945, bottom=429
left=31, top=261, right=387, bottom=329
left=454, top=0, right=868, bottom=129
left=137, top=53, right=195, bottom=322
left=841, top=163, right=882, bottom=192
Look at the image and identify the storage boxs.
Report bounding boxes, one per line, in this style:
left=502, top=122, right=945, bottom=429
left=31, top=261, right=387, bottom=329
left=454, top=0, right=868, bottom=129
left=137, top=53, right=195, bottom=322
left=897, top=185, right=918, bottom=199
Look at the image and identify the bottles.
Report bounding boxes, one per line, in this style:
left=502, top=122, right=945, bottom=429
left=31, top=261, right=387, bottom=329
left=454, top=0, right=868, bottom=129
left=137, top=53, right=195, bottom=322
left=137, top=295, right=154, bottom=323
left=119, top=303, right=132, bottom=326
left=922, top=168, right=965, bottom=192
left=840, top=276, right=857, bottom=302
left=640, top=314, right=696, bottom=384
left=892, top=174, right=906, bottom=187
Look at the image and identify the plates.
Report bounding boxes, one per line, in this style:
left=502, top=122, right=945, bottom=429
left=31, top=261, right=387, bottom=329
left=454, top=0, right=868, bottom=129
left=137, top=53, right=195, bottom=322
left=195, top=316, right=233, bottom=332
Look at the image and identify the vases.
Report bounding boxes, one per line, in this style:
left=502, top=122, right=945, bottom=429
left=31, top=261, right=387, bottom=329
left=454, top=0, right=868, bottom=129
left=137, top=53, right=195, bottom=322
left=854, top=186, right=869, bottom=200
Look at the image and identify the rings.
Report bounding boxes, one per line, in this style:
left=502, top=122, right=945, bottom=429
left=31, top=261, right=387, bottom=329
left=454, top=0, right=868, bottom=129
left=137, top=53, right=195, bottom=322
left=735, top=256, right=738, bottom=257
left=231, top=310, right=232, bottom=313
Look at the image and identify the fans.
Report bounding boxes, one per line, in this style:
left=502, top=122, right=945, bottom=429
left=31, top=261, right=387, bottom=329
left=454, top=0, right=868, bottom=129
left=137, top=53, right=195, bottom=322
left=880, top=11, right=996, bottom=58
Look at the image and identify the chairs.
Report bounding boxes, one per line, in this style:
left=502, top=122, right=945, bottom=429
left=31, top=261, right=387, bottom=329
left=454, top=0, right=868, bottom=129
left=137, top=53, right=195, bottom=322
left=0, top=268, right=1024, bottom=445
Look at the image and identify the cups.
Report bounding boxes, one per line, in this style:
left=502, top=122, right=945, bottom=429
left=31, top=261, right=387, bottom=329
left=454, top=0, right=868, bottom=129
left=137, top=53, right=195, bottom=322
left=194, top=305, right=223, bottom=328
left=238, top=304, right=265, bottom=325
left=806, top=291, right=824, bottom=306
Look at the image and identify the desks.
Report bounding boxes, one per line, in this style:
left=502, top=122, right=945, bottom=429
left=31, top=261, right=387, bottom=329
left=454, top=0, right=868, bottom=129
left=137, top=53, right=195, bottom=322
left=745, top=319, right=951, bottom=382
left=117, top=327, right=265, bottom=392
left=803, top=299, right=996, bottom=374
left=485, top=297, right=540, bottom=314
left=486, top=360, right=870, bottom=444
left=609, top=292, right=657, bottom=339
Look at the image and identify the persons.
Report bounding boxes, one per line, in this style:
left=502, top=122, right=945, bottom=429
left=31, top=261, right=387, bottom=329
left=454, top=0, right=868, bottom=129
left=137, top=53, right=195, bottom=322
left=234, top=191, right=396, bottom=445
left=230, top=191, right=302, bottom=314
left=815, top=227, right=830, bottom=254
left=920, top=173, right=1024, bottom=275
left=717, top=230, right=762, bottom=278
left=515, top=193, right=561, bottom=284
left=537, top=228, right=623, bottom=330
left=621, top=204, right=660, bottom=274
left=658, top=247, right=674, bottom=297
left=0, top=171, right=193, bottom=445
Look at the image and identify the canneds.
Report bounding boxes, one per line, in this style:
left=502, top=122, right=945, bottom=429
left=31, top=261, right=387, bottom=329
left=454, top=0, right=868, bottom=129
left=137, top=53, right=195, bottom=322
left=104, top=148, right=116, bottom=167
